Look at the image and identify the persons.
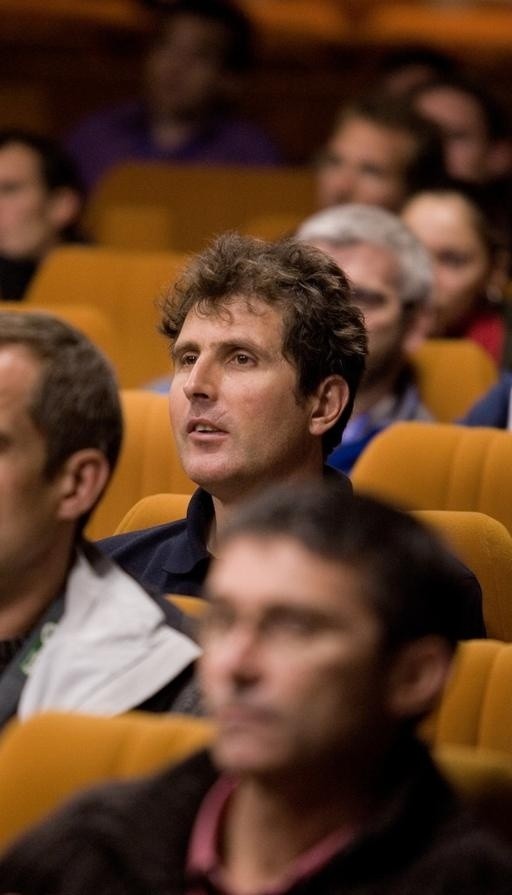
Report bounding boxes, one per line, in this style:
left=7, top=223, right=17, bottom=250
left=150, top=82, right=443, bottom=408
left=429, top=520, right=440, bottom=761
left=87, top=230, right=485, bottom=668
left=1, top=476, right=511, bottom=894
left=0, top=307, right=219, bottom=737
left=1, top=0, right=512, bottom=482
